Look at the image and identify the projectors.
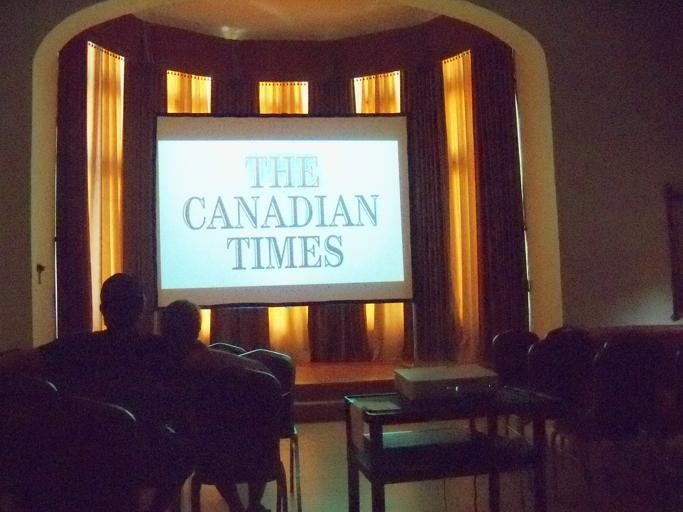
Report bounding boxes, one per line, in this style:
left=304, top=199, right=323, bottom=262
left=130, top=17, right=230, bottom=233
left=393, top=364, right=499, bottom=401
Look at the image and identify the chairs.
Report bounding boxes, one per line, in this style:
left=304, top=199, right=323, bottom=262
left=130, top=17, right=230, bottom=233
left=0, top=338, right=302, bottom=512
left=470, top=323, right=681, bottom=511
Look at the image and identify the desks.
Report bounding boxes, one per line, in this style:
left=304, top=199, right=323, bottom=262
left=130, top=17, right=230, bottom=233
left=341, top=386, right=567, bottom=510
